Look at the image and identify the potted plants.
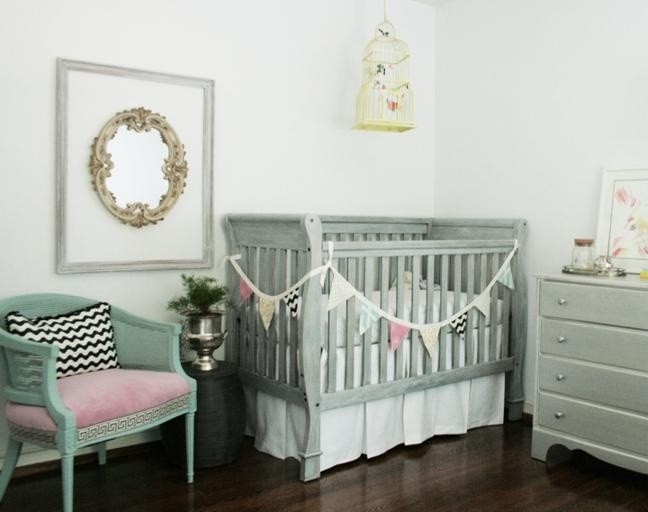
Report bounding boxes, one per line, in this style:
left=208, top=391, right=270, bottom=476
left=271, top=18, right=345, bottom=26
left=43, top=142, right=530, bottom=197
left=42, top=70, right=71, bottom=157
left=167, top=272, right=232, bottom=373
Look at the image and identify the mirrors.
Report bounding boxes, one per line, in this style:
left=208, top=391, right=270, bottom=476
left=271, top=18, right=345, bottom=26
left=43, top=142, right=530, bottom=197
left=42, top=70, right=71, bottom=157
left=54, top=55, right=216, bottom=275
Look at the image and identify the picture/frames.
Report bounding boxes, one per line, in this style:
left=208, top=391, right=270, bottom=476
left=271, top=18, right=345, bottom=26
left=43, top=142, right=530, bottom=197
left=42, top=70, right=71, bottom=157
left=597, top=169, right=647, bottom=271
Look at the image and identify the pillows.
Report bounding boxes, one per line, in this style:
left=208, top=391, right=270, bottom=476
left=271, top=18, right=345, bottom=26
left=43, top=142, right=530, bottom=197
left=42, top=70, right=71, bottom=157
left=5, top=302, right=122, bottom=379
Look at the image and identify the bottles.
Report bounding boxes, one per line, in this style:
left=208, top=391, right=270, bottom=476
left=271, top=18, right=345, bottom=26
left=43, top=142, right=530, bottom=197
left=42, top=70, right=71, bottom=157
left=572, top=238, right=595, bottom=271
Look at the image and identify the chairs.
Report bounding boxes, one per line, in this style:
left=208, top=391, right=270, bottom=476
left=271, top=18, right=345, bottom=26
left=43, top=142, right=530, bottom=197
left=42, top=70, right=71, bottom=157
left=0, top=293, right=198, bottom=512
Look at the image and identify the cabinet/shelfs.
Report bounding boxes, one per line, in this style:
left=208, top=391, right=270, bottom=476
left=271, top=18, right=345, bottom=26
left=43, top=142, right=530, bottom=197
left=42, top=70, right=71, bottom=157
left=530, top=265, right=648, bottom=476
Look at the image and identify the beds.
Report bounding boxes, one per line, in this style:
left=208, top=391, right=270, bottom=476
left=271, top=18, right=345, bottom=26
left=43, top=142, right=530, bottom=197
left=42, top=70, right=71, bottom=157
left=225, top=212, right=528, bottom=483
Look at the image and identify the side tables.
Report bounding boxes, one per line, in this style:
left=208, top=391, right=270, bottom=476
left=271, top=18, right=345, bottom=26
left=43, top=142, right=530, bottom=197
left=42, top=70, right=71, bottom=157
left=162, top=356, right=250, bottom=467
